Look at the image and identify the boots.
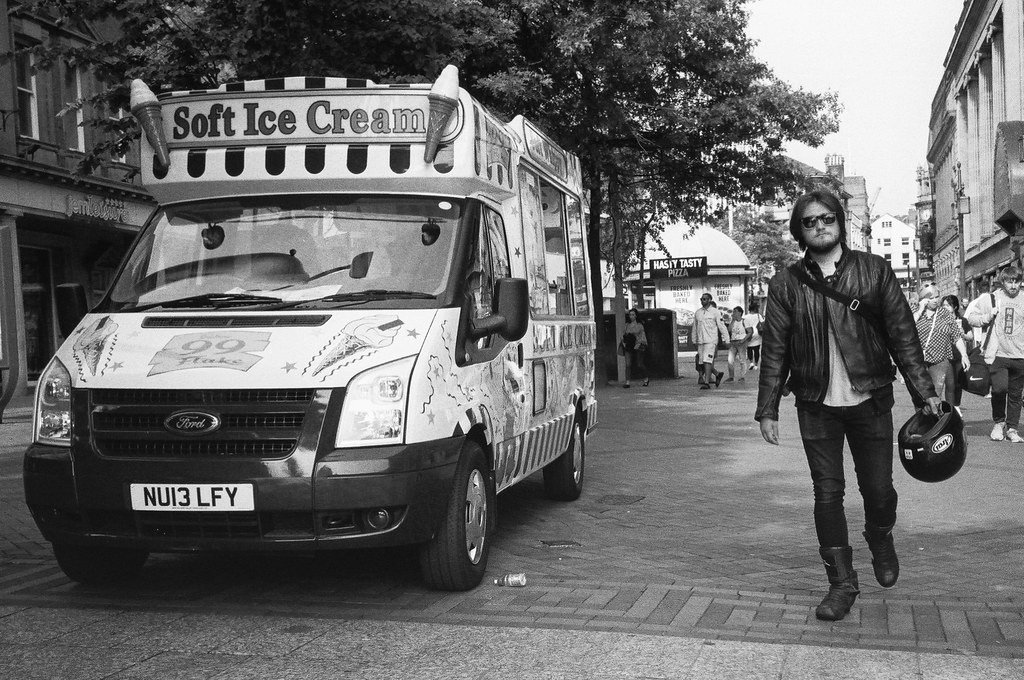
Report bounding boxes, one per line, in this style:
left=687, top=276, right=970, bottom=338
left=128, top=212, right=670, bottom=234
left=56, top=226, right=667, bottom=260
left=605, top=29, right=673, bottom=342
left=816, top=546, right=860, bottom=620
left=863, top=513, right=901, bottom=588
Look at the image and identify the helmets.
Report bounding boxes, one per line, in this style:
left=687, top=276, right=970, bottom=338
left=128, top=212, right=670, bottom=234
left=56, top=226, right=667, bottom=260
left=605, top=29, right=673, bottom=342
left=898, top=401, right=967, bottom=483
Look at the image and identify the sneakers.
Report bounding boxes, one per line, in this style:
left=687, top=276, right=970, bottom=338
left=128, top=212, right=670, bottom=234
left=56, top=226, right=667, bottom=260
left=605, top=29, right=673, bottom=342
left=990, top=423, right=1024, bottom=443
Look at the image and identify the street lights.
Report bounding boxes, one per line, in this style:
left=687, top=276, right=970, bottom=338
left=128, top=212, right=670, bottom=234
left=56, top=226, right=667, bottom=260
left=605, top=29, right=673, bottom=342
left=913, top=238, right=922, bottom=292
left=951, top=195, right=972, bottom=312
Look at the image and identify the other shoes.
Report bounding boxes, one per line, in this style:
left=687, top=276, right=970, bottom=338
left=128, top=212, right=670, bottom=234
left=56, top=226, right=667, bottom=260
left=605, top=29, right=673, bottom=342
left=724, top=378, right=734, bottom=383
left=700, top=372, right=724, bottom=389
left=698, top=377, right=716, bottom=384
left=642, top=379, right=650, bottom=387
left=623, top=383, right=631, bottom=388
left=749, top=363, right=758, bottom=370
left=738, top=378, right=745, bottom=383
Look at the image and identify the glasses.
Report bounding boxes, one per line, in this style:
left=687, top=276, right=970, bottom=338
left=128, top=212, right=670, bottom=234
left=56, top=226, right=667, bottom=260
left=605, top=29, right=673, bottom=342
left=800, top=212, right=837, bottom=229
left=919, top=282, right=936, bottom=293
left=700, top=298, right=707, bottom=301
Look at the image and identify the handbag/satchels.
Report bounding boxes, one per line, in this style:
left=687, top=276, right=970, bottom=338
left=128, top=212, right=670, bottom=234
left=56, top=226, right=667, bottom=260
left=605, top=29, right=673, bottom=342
left=958, top=346, right=990, bottom=396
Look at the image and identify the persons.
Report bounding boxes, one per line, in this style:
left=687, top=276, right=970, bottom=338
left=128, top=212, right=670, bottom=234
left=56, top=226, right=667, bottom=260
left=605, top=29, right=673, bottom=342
left=692, top=293, right=764, bottom=389
left=907, top=266, right=1024, bottom=443
left=621, top=308, right=650, bottom=388
left=754, top=190, right=944, bottom=620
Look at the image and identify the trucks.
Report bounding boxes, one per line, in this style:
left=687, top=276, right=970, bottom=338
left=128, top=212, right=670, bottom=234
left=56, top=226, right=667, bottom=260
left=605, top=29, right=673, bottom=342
left=22, top=63, right=599, bottom=591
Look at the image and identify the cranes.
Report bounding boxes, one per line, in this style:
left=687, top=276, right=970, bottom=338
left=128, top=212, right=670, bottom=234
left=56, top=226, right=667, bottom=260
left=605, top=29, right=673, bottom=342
left=862, top=186, right=882, bottom=252
